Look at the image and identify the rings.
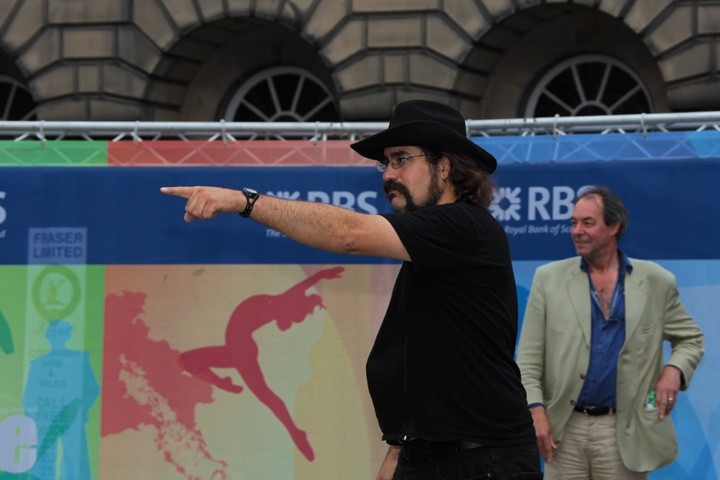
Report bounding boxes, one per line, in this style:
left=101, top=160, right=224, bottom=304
left=668, top=398, right=674, bottom=401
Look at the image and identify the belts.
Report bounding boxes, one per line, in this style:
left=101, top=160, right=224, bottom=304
left=574, top=406, right=615, bottom=417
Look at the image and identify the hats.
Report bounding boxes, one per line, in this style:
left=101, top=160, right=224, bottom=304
left=348, top=98, right=498, bottom=177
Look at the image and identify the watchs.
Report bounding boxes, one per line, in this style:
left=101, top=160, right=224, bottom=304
left=239, top=187, right=259, bottom=218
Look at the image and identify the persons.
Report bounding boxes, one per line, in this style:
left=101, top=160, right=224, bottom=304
left=159, top=98, right=543, bottom=480
left=515, top=188, right=706, bottom=480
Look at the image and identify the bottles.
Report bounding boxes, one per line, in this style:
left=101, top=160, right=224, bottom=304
left=644, top=390, right=656, bottom=410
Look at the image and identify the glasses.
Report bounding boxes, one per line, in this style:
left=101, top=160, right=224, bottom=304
left=375, top=153, right=425, bottom=173
